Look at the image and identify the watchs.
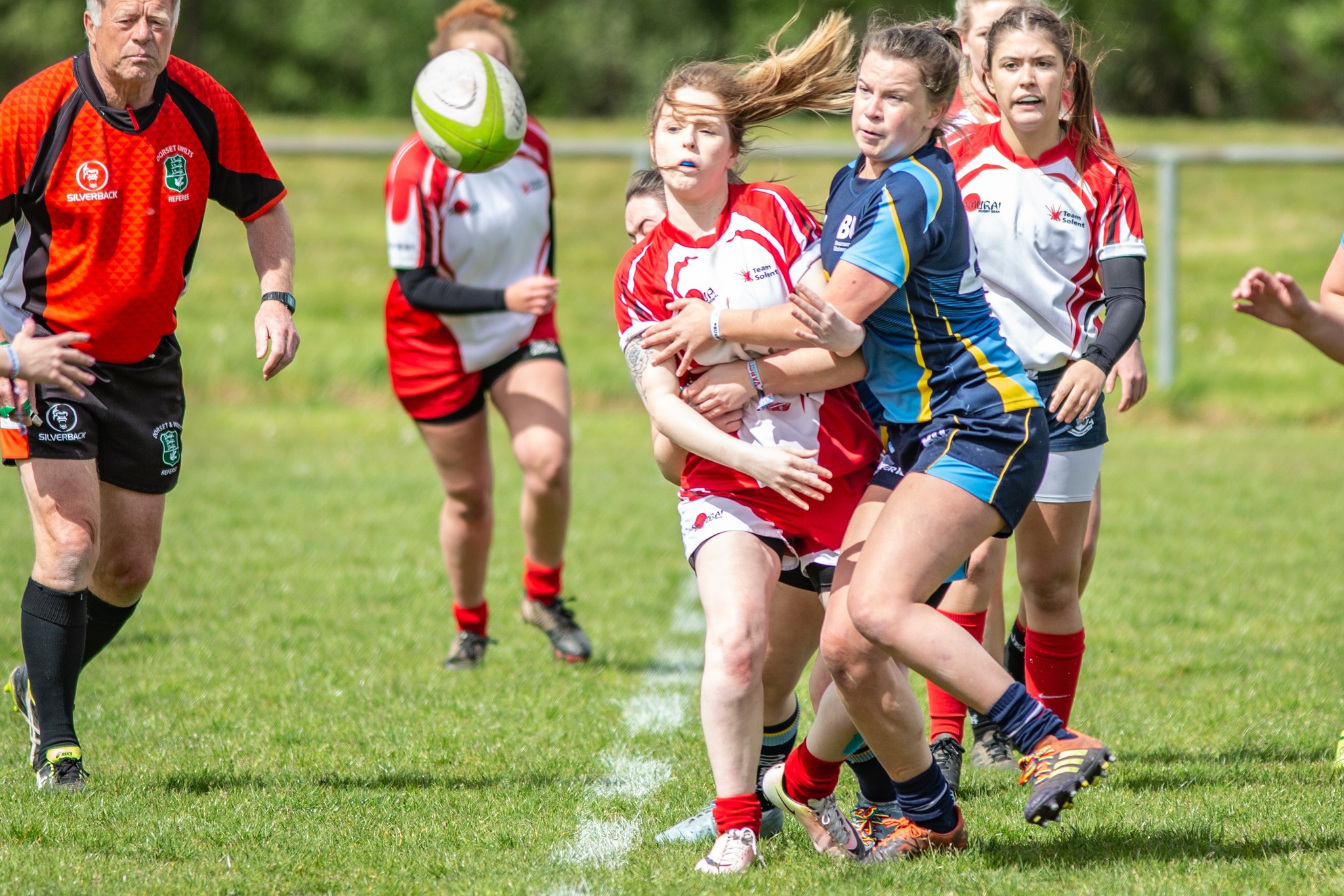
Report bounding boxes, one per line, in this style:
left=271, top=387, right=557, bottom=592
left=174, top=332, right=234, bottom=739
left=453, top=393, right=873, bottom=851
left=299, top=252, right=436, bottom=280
left=261, top=291, right=297, bottom=315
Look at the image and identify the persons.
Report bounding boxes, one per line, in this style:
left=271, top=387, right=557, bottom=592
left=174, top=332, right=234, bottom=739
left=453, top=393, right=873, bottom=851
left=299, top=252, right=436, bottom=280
left=0, top=0, right=302, bottom=784
left=0, top=318, right=95, bottom=400
left=382, top=0, right=597, bottom=665
left=1232, top=232, right=1344, bottom=365
left=614, top=0, right=1151, bottom=879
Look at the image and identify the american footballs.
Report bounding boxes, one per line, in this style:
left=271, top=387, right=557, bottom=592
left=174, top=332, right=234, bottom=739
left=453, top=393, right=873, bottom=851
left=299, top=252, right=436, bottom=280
left=412, top=48, right=527, bottom=173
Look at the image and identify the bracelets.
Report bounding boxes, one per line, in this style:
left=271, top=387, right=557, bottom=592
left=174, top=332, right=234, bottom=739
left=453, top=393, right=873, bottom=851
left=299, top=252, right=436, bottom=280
left=746, top=359, right=764, bottom=394
left=711, top=306, right=725, bottom=341
left=4, top=343, right=20, bottom=380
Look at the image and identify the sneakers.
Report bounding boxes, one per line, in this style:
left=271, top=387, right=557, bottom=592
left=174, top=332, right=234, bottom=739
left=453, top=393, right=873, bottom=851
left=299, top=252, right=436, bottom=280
left=1017, top=725, right=1117, bottom=827
left=862, top=805, right=967, bottom=866
left=762, top=762, right=865, bottom=863
left=694, top=826, right=766, bottom=876
left=929, top=733, right=966, bottom=791
left=35, top=744, right=91, bottom=795
left=656, top=796, right=784, bottom=843
left=971, top=729, right=1020, bottom=770
left=3, top=664, right=40, bottom=744
left=441, top=629, right=499, bottom=668
left=851, top=791, right=903, bottom=851
left=521, top=594, right=593, bottom=661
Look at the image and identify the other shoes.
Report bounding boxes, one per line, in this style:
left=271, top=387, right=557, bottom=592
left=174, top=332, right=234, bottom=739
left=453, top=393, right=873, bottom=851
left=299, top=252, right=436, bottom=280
left=1334, top=728, right=1344, bottom=764
